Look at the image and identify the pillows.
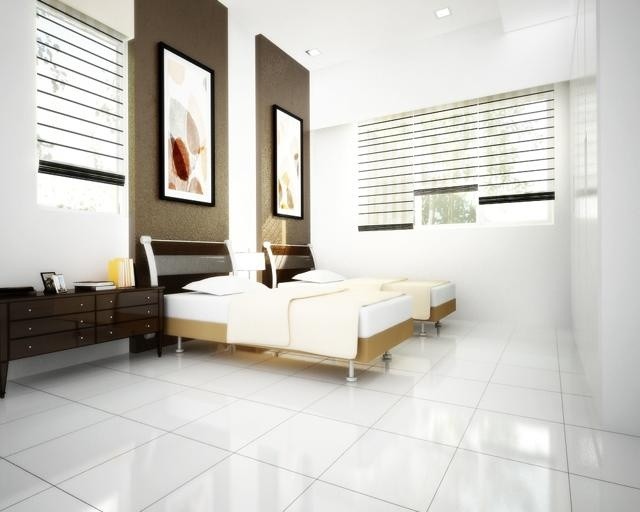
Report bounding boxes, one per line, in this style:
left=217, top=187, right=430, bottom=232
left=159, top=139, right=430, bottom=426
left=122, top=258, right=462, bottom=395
left=181, top=275, right=270, bottom=296
left=291, top=269, right=344, bottom=283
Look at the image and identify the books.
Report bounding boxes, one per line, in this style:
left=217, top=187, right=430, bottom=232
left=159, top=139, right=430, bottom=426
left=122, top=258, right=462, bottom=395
left=75, top=286, right=117, bottom=291
left=71, top=280, right=115, bottom=286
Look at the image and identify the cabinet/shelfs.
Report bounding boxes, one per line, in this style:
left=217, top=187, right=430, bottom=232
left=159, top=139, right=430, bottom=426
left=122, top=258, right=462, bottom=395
left=1, top=287, right=167, bottom=398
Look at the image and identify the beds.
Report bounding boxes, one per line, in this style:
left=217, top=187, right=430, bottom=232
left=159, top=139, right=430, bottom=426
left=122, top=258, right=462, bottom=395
left=140, top=235, right=413, bottom=382
left=264, top=242, right=456, bottom=336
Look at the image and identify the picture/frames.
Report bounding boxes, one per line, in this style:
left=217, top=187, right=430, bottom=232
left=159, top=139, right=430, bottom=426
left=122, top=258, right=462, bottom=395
left=158, top=42, right=215, bottom=207
left=272, top=104, right=303, bottom=220
left=40, top=272, right=55, bottom=295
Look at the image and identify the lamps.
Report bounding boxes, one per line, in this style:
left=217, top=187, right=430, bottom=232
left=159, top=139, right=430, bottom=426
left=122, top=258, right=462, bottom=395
left=234, top=248, right=266, bottom=280
